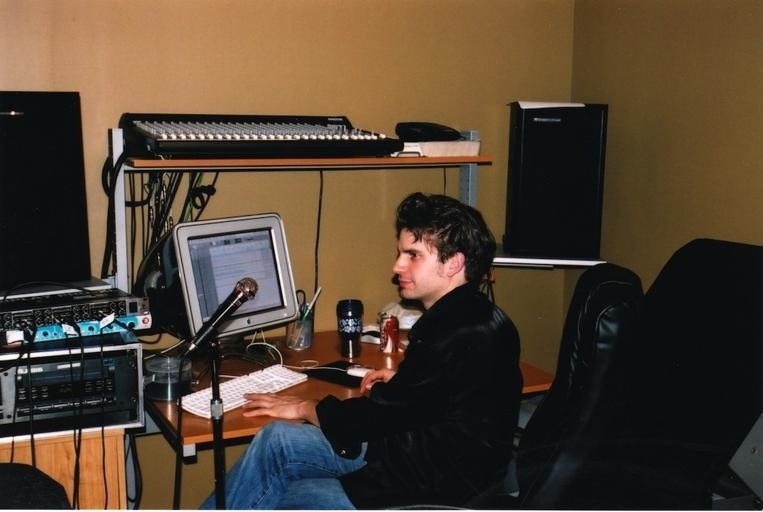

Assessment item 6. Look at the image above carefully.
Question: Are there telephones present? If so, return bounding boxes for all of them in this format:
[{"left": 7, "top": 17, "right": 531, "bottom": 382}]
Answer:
[{"left": 396, "top": 122, "right": 461, "bottom": 141}]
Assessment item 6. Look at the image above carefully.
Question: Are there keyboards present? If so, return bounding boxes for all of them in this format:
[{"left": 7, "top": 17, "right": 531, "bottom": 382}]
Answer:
[{"left": 176, "top": 364, "right": 308, "bottom": 420}]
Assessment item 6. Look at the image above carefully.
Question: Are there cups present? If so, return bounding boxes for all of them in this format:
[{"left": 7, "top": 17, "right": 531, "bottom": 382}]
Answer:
[
  {"left": 336, "top": 300, "right": 364, "bottom": 357},
  {"left": 287, "top": 321, "right": 312, "bottom": 351}
]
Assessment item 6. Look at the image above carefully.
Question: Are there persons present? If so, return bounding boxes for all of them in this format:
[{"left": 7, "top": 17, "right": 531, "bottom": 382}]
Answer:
[{"left": 186, "top": 191, "right": 525, "bottom": 510}]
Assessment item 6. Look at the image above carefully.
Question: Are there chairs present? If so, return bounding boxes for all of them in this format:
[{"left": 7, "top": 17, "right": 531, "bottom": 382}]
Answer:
[
  {"left": 380, "top": 264, "right": 644, "bottom": 509},
  {"left": 594, "top": 237, "right": 763, "bottom": 510}
]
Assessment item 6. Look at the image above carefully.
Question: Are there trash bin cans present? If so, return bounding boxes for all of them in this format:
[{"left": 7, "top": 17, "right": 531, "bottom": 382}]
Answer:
[{"left": 0, "top": 463, "right": 74, "bottom": 509}]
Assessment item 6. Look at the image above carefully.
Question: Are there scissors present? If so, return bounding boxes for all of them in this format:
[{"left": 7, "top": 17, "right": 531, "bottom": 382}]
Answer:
[{"left": 296, "top": 289, "right": 306, "bottom": 322}]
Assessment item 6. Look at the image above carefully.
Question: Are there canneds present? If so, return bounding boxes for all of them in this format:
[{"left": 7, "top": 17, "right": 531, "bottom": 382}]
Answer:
[{"left": 380, "top": 315, "right": 399, "bottom": 353}]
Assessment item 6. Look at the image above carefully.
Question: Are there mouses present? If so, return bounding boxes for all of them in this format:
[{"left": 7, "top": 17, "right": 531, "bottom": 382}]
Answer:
[{"left": 345, "top": 367, "right": 376, "bottom": 379}]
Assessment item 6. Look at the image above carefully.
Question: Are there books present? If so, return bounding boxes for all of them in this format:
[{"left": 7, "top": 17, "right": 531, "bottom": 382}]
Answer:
[{"left": 389, "top": 139, "right": 482, "bottom": 158}]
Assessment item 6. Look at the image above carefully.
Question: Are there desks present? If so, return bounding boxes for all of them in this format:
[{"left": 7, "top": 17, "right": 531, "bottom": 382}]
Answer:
[{"left": 141, "top": 324, "right": 555, "bottom": 509}]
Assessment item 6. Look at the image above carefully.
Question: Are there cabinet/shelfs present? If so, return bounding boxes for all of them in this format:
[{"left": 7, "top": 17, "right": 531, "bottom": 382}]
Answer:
[{"left": 0, "top": 429, "right": 129, "bottom": 509}]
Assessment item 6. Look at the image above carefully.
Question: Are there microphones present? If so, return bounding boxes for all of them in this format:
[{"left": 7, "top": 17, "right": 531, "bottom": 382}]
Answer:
[{"left": 183, "top": 276, "right": 259, "bottom": 358}]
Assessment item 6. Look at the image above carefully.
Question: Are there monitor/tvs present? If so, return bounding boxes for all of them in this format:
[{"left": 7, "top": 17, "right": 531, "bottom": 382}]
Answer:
[
  {"left": 134, "top": 211, "right": 301, "bottom": 342},
  {"left": 0, "top": 90, "right": 92, "bottom": 293}
]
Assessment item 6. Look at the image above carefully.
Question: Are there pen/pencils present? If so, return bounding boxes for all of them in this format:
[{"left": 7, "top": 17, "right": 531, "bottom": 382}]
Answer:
[{"left": 302, "top": 286, "right": 323, "bottom": 321}]
